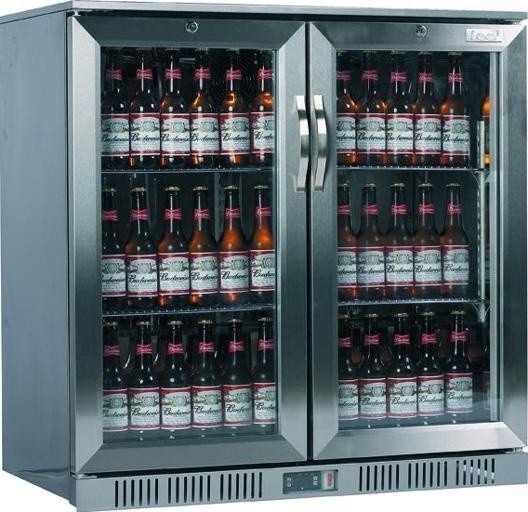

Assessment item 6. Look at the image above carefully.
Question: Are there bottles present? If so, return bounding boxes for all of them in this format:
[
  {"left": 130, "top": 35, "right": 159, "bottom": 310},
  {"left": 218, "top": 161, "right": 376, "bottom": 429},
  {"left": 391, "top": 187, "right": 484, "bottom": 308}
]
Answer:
[
  {"left": 439, "top": 184, "right": 471, "bottom": 300},
  {"left": 129, "top": 321, "right": 159, "bottom": 434},
  {"left": 123, "top": 188, "right": 158, "bottom": 311},
  {"left": 100, "top": 47, "right": 130, "bottom": 170},
  {"left": 416, "top": 311, "right": 445, "bottom": 420},
  {"left": 384, "top": 182, "right": 414, "bottom": 300},
  {"left": 102, "top": 319, "right": 129, "bottom": 440},
  {"left": 386, "top": 50, "right": 415, "bottom": 168},
  {"left": 158, "top": 47, "right": 192, "bottom": 170},
  {"left": 440, "top": 51, "right": 471, "bottom": 168},
  {"left": 102, "top": 187, "right": 127, "bottom": 312},
  {"left": 337, "top": 315, "right": 359, "bottom": 424},
  {"left": 481, "top": 71, "right": 490, "bottom": 166},
  {"left": 359, "top": 312, "right": 386, "bottom": 422},
  {"left": 337, "top": 179, "right": 358, "bottom": 300},
  {"left": 160, "top": 319, "right": 192, "bottom": 429},
  {"left": 222, "top": 319, "right": 255, "bottom": 430},
  {"left": 189, "top": 47, "right": 220, "bottom": 168},
  {"left": 129, "top": 46, "right": 162, "bottom": 171},
  {"left": 250, "top": 50, "right": 274, "bottom": 166},
  {"left": 220, "top": 187, "right": 252, "bottom": 309},
  {"left": 388, "top": 312, "right": 417, "bottom": 419},
  {"left": 250, "top": 185, "right": 276, "bottom": 305},
  {"left": 443, "top": 310, "right": 473, "bottom": 418},
  {"left": 337, "top": 51, "right": 357, "bottom": 166},
  {"left": 220, "top": 47, "right": 251, "bottom": 168},
  {"left": 158, "top": 185, "right": 190, "bottom": 308},
  {"left": 413, "top": 51, "right": 442, "bottom": 169},
  {"left": 412, "top": 184, "right": 442, "bottom": 300},
  {"left": 251, "top": 316, "right": 276, "bottom": 429},
  {"left": 357, "top": 182, "right": 387, "bottom": 301},
  {"left": 355, "top": 50, "right": 386, "bottom": 168},
  {"left": 187, "top": 186, "right": 219, "bottom": 310},
  {"left": 193, "top": 319, "right": 223, "bottom": 430}
]
